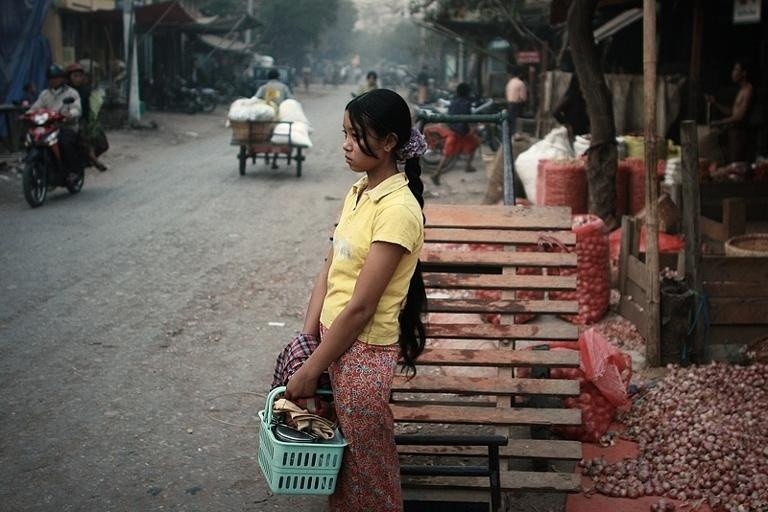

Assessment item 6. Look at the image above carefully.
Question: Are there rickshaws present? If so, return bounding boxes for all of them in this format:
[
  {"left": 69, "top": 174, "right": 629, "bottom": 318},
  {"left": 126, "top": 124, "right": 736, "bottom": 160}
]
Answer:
[{"left": 386, "top": 108, "right": 585, "bottom": 510}]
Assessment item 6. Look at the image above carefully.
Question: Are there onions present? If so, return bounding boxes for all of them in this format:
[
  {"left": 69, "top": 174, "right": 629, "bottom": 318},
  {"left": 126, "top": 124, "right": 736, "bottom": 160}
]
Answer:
[{"left": 470, "top": 213, "right": 611, "bottom": 326}]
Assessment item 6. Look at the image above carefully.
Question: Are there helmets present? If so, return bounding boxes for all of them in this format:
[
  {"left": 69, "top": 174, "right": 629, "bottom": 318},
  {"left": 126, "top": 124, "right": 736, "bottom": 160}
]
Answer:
[{"left": 46, "top": 64, "right": 83, "bottom": 77}]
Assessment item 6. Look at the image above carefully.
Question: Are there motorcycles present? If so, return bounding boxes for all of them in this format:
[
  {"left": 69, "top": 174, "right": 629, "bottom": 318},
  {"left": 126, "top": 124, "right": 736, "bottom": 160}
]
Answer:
[
  {"left": 404, "top": 74, "right": 501, "bottom": 172},
  {"left": 172, "top": 75, "right": 217, "bottom": 114},
  {"left": 142, "top": 80, "right": 200, "bottom": 114},
  {"left": 7, "top": 96, "right": 89, "bottom": 209}
]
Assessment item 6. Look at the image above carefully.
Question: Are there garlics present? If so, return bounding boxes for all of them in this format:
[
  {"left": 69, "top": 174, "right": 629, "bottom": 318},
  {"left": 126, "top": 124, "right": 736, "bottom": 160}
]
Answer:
[{"left": 515, "top": 340, "right": 767, "bottom": 512}]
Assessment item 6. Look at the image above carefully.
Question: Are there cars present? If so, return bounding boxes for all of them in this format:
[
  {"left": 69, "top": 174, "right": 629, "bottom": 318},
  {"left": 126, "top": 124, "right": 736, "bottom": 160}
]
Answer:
[{"left": 250, "top": 65, "right": 291, "bottom": 96}]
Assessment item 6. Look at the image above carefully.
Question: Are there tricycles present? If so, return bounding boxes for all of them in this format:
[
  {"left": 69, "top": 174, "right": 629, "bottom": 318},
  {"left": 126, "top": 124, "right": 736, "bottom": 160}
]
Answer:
[{"left": 235, "top": 120, "right": 308, "bottom": 179}]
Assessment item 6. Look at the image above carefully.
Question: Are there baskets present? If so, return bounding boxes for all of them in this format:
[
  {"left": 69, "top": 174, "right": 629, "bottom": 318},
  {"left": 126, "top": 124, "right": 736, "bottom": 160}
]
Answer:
[
  {"left": 258, "top": 386, "right": 348, "bottom": 495},
  {"left": 230, "top": 121, "right": 272, "bottom": 142},
  {"left": 725, "top": 233, "right": 768, "bottom": 258}
]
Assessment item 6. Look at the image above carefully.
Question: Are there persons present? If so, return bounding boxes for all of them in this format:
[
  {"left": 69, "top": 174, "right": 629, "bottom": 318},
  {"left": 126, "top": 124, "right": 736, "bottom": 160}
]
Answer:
[
  {"left": 356, "top": 68, "right": 380, "bottom": 96},
  {"left": 415, "top": 59, "right": 429, "bottom": 107},
  {"left": 66, "top": 63, "right": 110, "bottom": 171},
  {"left": 283, "top": 87, "right": 434, "bottom": 512},
  {"left": 550, "top": 77, "right": 589, "bottom": 144},
  {"left": 703, "top": 57, "right": 755, "bottom": 163},
  {"left": 504, "top": 66, "right": 528, "bottom": 130},
  {"left": 250, "top": 68, "right": 296, "bottom": 171},
  {"left": 429, "top": 80, "right": 479, "bottom": 187},
  {"left": 26, "top": 63, "right": 83, "bottom": 186},
  {"left": 301, "top": 59, "right": 312, "bottom": 96}
]
